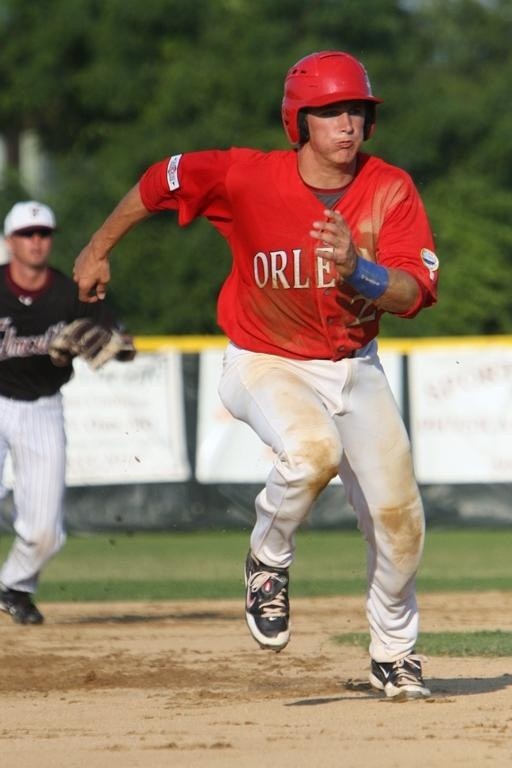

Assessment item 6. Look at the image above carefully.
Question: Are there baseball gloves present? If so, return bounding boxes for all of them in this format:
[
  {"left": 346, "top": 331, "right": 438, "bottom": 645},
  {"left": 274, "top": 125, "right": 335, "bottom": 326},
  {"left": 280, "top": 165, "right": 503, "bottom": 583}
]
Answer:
[{"left": 47, "top": 319, "right": 124, "bottom": 368}]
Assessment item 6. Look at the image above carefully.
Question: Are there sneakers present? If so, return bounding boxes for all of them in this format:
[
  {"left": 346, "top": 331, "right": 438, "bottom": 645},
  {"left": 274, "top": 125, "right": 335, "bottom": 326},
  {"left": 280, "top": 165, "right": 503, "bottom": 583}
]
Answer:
[
  {"left": 0, "top": 586, "right": 44, "bottom": 627},
  {"left": 366, "top": 653, "right": 430, "bottom": 700},
  {"left": 243, "top": 548, "right": 291, "bottom": 651}
]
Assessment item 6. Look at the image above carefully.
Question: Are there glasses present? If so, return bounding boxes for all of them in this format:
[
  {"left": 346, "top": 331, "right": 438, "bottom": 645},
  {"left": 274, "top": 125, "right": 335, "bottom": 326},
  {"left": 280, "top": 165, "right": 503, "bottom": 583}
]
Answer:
[{"left": 14, "top": 227, "right": 54, "bottom": 239}]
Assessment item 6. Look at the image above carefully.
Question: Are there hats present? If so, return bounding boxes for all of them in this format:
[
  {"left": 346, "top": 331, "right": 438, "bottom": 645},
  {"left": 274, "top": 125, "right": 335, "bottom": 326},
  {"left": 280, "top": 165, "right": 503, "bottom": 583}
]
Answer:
[
  {"left": 281, "top": 49, "right": 383, "bottom": 146},
  {"left": 4, "top": 201, "right": 58, "bottom": 240}
]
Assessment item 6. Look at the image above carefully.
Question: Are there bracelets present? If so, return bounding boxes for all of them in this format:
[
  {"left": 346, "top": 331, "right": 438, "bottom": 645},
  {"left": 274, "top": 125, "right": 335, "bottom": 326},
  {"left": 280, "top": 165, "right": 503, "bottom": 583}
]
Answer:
[{"left": 343, "top": 254, "right": 389, "bottom": 301}]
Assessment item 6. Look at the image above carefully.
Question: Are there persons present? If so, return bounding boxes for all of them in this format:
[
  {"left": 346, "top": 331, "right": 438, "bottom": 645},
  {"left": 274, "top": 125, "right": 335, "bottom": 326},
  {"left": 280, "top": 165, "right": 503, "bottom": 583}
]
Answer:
[
  {"left": 72, "top": 50, "right": 440, "bottom": 699},
  {"left": 0, "top": 202, "right": 136, "bottom": 626}
]
